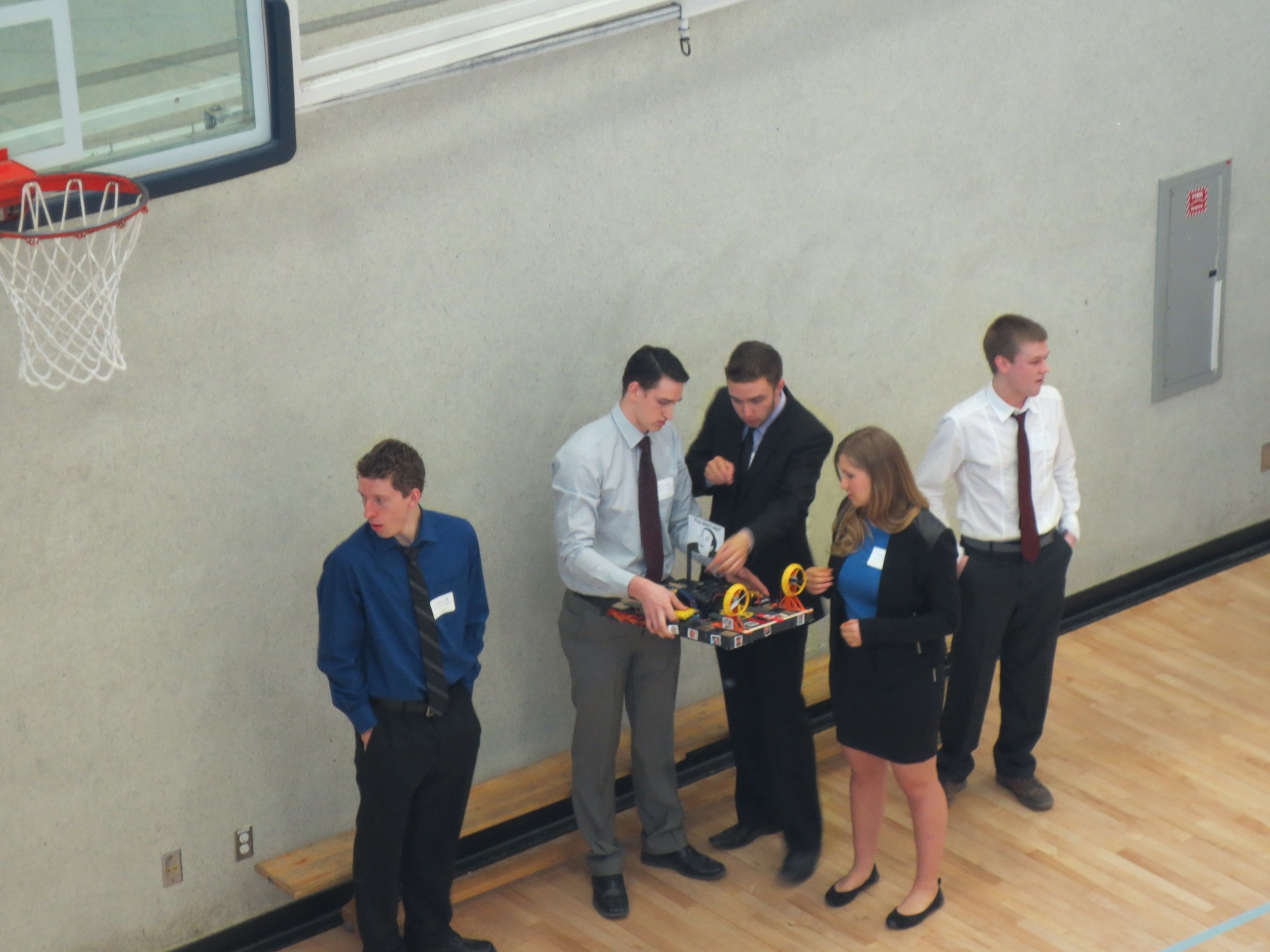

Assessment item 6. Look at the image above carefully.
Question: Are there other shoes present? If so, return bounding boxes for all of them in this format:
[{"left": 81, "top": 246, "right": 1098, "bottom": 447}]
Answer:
[
  {"left": 430, "top": 934, "right": 493, "bottom": 952},
  {"left": 886, "top": 879, "right": 945, "bottom": 929},
  {"left": 942, "top": 780, "right": 965, "bottom": 796},
  {"left": 997, "top": 774, "right": 1052, "bottom": 810},
  {"left": 825, "top": 865, "right": 880, "bottom": 907}
]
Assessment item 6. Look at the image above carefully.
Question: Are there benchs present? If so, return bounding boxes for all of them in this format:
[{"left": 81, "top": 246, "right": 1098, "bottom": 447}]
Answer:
[{"left": 255, "top": 656, "right": 829, "bottom": 926}]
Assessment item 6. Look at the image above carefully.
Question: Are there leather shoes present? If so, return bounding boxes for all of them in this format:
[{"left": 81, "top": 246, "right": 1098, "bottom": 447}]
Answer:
[
  {"left": 641, "top": 843, "right": 726, "bottom": 880},
  {"left": 710, "top": 822, "right": 782, "bottom": 848},
  {"left": 780, "top": 848, "right": 818, "bottom": 881},
  {"left": 592, "top": 873, "right": 630, "bottom": 917}
]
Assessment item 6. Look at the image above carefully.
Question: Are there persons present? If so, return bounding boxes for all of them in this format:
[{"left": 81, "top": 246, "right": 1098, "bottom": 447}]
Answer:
[
  {"left": 679, "top": 341, "right": 832, "bottom": 884},
  {"left": 920, "top": 315, "right": 1083, "bottom": 808},
  {"left": 316, "top": 439, "right": 497, "bottom": 952},
  {"left": 807, "top": 426, "right": 962, "bottom": 931},
  {"left": 550, "top": 345, "right": 769, "bottom": 920},
  {"left": 686, "top": 529, "right": 717, "bottom": 558}
]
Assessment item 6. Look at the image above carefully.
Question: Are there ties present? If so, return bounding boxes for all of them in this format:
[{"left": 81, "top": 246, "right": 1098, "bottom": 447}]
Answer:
[
  {"left": 737, "top": 429, "right": 755, "bottom": 486},
  {"left": 1012, "top": 411, "right": 1039, "bottom": 561},
  {"left": 637, "top": 436, "right": 663, "bottom": 582},
  {"left": 397, "top": 545, "right": 449, "bottom": 716}
]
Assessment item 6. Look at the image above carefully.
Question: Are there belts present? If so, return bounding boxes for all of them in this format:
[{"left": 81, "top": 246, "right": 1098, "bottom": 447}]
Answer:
[
  {"left": 962, "top": 529, "right": 1055, "bottom": 553},
  {"left": 376, "top": 685, "right": 463, "bottom": 717},
  {"left": 578, "top": 593, "right": 619, "bottom": 606}
]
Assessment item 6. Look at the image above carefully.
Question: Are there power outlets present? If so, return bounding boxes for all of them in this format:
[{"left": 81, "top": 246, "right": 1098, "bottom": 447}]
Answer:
[
  {"left": 234, "top": 824, "right": 254, "bottom": 862},
  {"left": 162, "top": 850, "right": 183, "bottom": 889}
]
